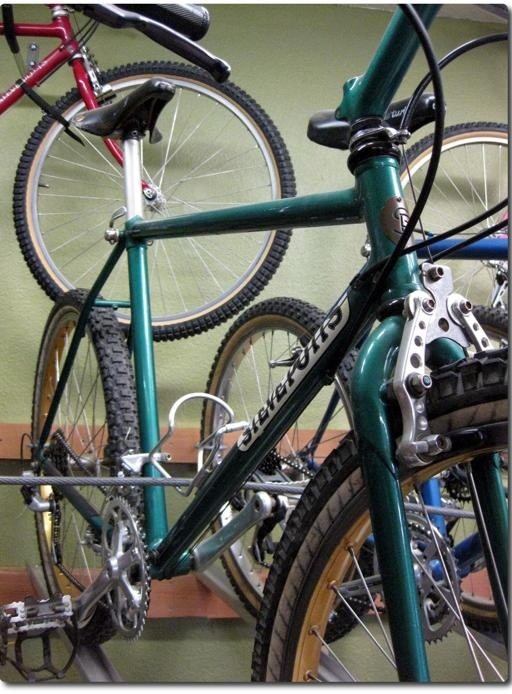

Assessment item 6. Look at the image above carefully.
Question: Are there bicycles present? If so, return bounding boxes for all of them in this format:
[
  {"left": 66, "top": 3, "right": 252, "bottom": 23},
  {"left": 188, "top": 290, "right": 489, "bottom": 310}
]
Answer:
[{"left": 2, "top": 4, "right": 510, "bottom": 683}]
